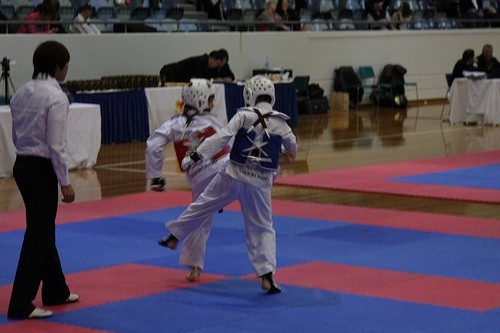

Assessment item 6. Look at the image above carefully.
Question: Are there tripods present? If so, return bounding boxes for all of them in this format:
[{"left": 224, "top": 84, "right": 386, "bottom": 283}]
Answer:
[{"left": 0, "top": 71, "right": 15, "bottom": 105}]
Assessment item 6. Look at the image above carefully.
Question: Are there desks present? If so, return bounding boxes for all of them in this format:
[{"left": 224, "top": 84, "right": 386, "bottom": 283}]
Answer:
[
  {"left": 0, "top": 103, "right": 102, "bottom": 178},
  {"left": 143, "top": 83, "right": 228, "bottom": 136},
  {"left": 447, "top": 78, "right": 500, "bottom": 125}
]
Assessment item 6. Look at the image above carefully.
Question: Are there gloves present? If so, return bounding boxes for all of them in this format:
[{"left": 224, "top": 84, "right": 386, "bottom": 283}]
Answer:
[
  {"left": 151, "top": 177, "right": 166, "bottom": 190},
  {"left": 179, "top": 149, "right": 204, "bottom": 171}
]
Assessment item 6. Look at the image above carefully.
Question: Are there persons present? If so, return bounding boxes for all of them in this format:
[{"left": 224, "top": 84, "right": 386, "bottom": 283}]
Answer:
[
  {"left": 18, "top": 0, "right": 500, "bottom": 37},
  {"left": 159, "top": 49, "right": 235, "bottom": 80},
  {"left": 5, "top": 40, "right": 79, "bottom": 319},
  {"left": 158, "top": 75, "right": 298, "bottom": 292},
  {"left": 450, "top": 44, "right": 500, "bottom": 87},
  {"left": 144, "top": 78, "right": 282, "bottom": 282}
]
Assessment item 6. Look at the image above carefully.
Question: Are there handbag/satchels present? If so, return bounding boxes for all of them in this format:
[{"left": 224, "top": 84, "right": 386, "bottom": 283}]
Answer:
[
  {"left": 304, "top": 97, "right": 330, "bottom": 112},
  {"left": 296, "top": 83, "right": 324, "bottom": 99}
]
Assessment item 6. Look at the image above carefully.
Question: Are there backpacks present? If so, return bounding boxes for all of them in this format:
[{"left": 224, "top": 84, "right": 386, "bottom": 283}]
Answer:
[
  {"left": 370, "top": 64, "right": 408, "bottom": 109},
  {"left": 334, "top": 66, "right": 361, "bottom": 92}
]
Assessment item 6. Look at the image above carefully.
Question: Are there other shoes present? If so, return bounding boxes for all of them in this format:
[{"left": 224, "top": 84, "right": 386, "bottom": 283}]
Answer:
[
  {"left": 7, "top": 308, "right": 53, "bottom": 319},
  {"left": 42, "top": 293, "right": 79, "bottom": 305}
]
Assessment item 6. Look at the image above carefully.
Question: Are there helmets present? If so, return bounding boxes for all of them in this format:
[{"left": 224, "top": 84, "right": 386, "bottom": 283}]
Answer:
[
  {"left": 243, "top": 75, "right": 276, "bottom": 107},
  {"left": 181, "top": 78, "right": 216, "bottom": 113}
]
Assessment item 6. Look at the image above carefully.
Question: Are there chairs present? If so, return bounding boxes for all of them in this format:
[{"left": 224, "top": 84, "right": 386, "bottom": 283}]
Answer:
[
  {"left": 212, "top": 0, "right": 462, "bottom": 31},
  {"left": 359, "top": 65, "right": 393, "bottom": 108},
  {"left": 97, "top": 6, "right": 198, "bottom": 32},
  {"left": 440, "top": 74, "right": 452, "bottom": 120},
  {"left": 294, "top": 76, "right": 313, "bottom": 113},
  {"left": 1, "top": 4, "right": 35, "bottom": 21},
  {"left": 60, "top": 6, "right": 76, "bottom": 30}
]
